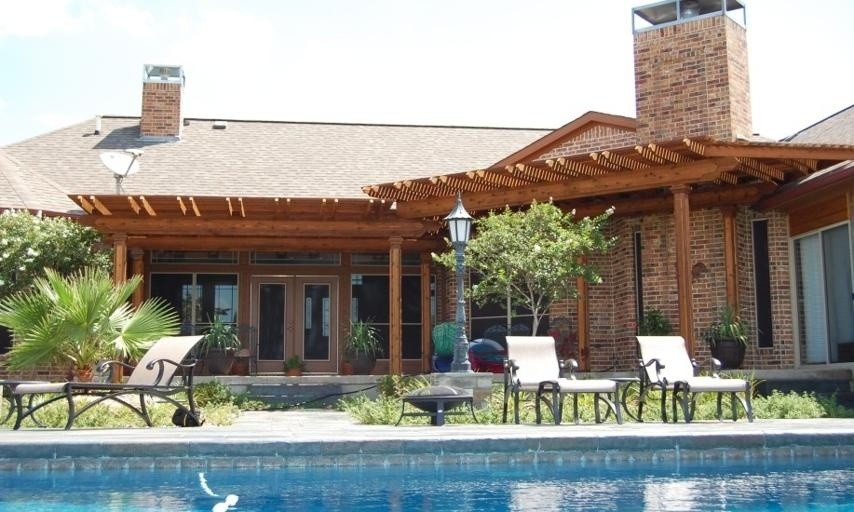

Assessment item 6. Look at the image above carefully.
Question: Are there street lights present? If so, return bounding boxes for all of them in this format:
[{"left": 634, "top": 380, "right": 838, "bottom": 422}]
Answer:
[{"left": 441, "top": 187, "right": 476, "bottom": 371}]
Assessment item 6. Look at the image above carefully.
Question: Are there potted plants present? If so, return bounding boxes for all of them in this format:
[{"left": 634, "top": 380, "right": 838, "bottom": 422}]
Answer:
[
  {"left": 195, "top": 311, "right": 241, "bottom": 374},
  {"left": 340, "top": 313, "right": 384, "bottom": 375},
  {"left": 282, "top": 356, "right": 308, "bottom": 376},
  {"left": 699, "top": 305, "right": 758, "bottom": 369}
]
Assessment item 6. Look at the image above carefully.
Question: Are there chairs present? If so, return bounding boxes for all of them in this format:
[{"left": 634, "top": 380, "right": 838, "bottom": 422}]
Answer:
[
  {"left": 640, "top": 470, "right": 853, "bottom": 512},
  {"left": 635, "top": 333, "right": 754, "bottom": 426},
  {"left": 508, "top": 477, "right": 623, "bottom": 512},
  {"left": 107, "top": 488, "right": 189, "bottom": 512},
  {"left": 12, "top": 333, "right": 206, "bottom": 431},
  {"left": 498, "top": 333, "right": 621, "bottom": 428}
]
